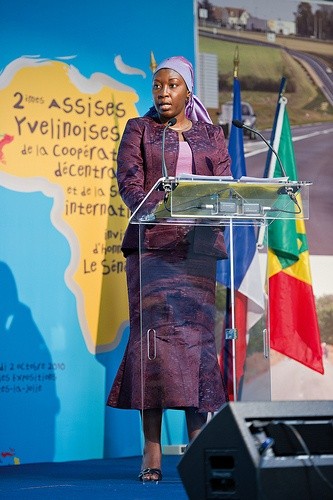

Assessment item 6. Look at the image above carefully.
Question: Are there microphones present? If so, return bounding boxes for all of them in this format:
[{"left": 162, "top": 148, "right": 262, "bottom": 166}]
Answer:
[
  {"left": 162, "top": 118, "right": 176, "bottom": 177},
  {"left": 232, "top": 120, "right": 286, "bottom": 177}
]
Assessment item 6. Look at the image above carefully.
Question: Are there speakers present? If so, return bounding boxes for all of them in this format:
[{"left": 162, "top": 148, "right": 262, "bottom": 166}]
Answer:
[{"left": 176, "top": 399, "right": 333, "bottom": 500}]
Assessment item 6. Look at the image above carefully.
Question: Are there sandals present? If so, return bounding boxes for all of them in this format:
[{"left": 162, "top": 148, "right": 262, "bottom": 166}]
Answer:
[{"left": 138, "top": 468, "right": 162, "bottom": 483}]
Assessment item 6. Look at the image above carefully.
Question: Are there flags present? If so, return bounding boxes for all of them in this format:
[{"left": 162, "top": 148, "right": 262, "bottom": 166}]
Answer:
[{"left": 217, "top": 70, "right": 325, "bottom": 403}]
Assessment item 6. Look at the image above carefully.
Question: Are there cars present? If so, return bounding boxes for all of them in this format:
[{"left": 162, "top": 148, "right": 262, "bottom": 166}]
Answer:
[{"left": 217, "top": 100, "right": 258, "bottom": 139}]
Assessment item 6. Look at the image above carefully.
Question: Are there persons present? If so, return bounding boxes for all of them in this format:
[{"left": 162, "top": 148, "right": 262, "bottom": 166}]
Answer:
[{"left": 104, "top": 54, "right": 233, "bottom": 483}]
